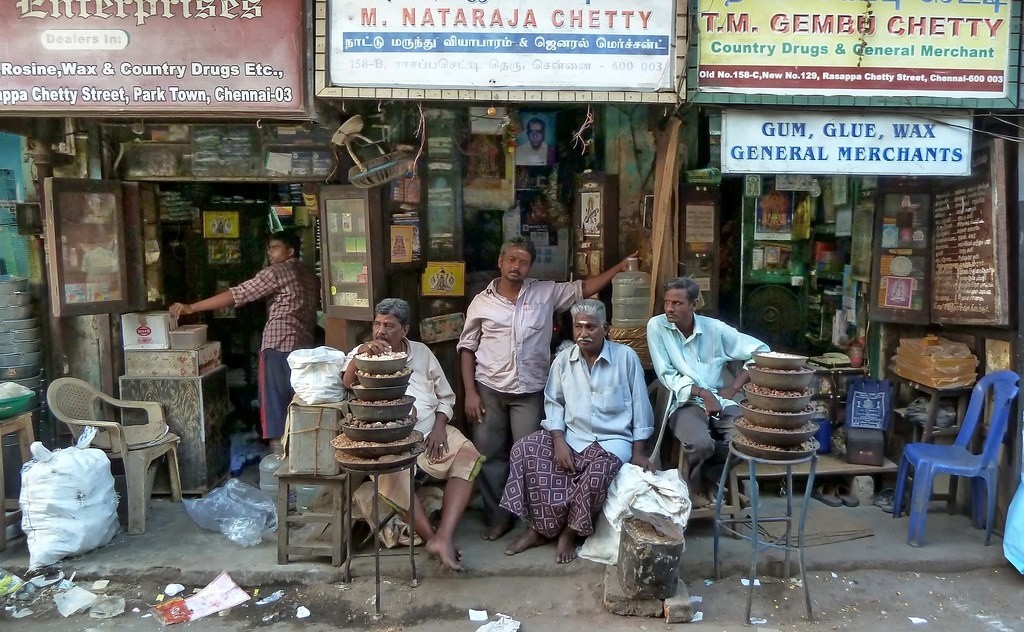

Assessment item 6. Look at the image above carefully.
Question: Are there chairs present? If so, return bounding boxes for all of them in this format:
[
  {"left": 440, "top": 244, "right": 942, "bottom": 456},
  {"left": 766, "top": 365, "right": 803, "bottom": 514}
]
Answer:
[
  {"left": 49, "top": 376, "right": 183, "bottom": 534},
  {"left": 643, "top": 379, "right": 675, "bottom": 468},
  {"left": 894, "top": 368, "right": 1019, "bottom": 547}
]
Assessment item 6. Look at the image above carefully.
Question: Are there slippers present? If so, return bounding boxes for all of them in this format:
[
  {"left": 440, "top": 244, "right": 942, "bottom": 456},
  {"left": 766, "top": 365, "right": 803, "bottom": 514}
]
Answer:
[
  {"left": 873, "top": 484, "right": 907, "bottom": 513},
  {"left": 810, "top": 480, "right": 860, "bottom": 507}
]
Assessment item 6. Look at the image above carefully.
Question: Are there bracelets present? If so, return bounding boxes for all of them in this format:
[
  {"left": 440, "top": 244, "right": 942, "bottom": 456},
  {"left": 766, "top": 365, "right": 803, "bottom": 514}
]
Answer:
[
  {"left": 552, "top": 435, "right": 563, "bottom": 442},
  {"left": 698, "top": 388, "right": 703, "bottom": 397}
]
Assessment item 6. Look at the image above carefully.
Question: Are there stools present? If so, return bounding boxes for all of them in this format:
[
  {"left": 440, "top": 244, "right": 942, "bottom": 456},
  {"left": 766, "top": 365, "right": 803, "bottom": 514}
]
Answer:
[
  {"left": 275, "top": 463, "right": 348, "bottom": 568},
  {"left": 0, "top": 412, "right": 37, "bottom": 551}
]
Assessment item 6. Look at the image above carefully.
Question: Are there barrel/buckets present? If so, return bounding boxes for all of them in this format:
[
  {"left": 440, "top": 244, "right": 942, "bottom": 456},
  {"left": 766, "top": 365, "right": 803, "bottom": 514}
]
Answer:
[
  {"left": 0, "top": 275, "right": 44, "bottom": 494},
  {"left": 812, "top": 417, "right": 831, "bottom": 455}
]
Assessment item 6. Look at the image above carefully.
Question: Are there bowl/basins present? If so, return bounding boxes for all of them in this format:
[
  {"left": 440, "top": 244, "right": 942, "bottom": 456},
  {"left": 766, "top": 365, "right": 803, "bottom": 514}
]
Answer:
[
  {"left": 0, "top": 392, "right": 36, "bottom": 419},
  {"left": 731, "top": 353, "right": 821, "bottom": 461},
  {"left": 329, "top": 352, "right": 431, "bottom": 470}
]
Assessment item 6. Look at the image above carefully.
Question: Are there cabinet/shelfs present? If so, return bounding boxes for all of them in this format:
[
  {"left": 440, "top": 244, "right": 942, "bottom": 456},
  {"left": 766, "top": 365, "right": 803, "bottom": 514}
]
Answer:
[
  {"left": 806, "top": 370, "right": 869, "bottom": 428},
  {"left": 342, "top": 457, "right": 423, "bottom": 621},
  {"left": 886, "top": 373, "right": 978, "bottom": 516},
  {"left": 48, "top": 121, "right": 382, "bottom": 320},
  {"left": 714, "top": 439, "right": 818, "bottom": 625},
  {"left": 742, "top": 178, "right": 932, "bottom": 365}
]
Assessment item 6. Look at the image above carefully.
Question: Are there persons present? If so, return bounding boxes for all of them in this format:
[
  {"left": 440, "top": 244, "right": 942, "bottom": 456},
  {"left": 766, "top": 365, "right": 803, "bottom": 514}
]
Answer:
[
  {"left": 504, "top": 299, "right": 657, "bottom": 564},
  {"left": 341, "top": 297, "right": 486, "bottom": 574},
  {"left": 169, "top": 231, "right": 318, "bottom": 454},
  {"left": 457, "top": 238, "right": 644, "bottom": 541},
  {"left": 646, "top": 277, "right": 771, "bottom": 507},
  {"left": 516, "top": 117, "right": 555, "bottom": 165}
]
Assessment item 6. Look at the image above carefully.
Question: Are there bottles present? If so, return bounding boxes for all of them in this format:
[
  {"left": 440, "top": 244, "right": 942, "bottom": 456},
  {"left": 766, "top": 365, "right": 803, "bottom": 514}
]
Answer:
[
  {"left": 611, "top": 258, "right": 651, "bottom": 328},
  {"left": 258, "top": 440, "right": 326, "bottom": 514},
  {"left": 849, "top": 342, "right": 863, "bottom": 368}
]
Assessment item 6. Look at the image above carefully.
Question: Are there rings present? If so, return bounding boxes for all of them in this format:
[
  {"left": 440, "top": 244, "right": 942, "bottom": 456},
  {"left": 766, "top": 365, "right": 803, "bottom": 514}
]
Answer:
[
  {"left": 367, "top": 344, "right": 372, "bottom": 348},
  {"left": 439, "top": 444, "right": 444, "bottom": 448}
]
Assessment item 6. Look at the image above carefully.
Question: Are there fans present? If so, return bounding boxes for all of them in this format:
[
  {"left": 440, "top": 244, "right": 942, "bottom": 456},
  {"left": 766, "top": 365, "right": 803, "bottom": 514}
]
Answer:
[
  {"left": 331, "top": 112, "right": 410, "bottom": 189},
  {"left": 744, "top": 285, "right": 802, "bottom": 341}
]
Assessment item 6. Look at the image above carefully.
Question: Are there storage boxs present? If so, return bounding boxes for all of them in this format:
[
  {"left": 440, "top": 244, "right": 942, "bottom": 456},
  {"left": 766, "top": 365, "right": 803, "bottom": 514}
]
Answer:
[
  {"left": 123, "top": 309, "right": 178, "bottom": 350},
  {"left": 125, "top": 341, "right": 221, "bottom": 378},
  {"left": 169, "top": 322, "right": 209, "bottom": 349}
]
